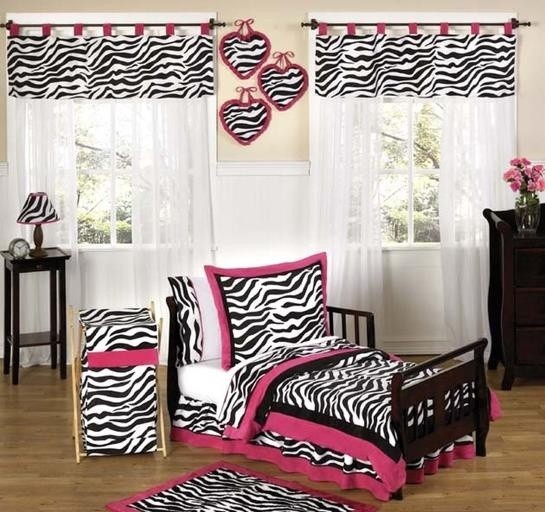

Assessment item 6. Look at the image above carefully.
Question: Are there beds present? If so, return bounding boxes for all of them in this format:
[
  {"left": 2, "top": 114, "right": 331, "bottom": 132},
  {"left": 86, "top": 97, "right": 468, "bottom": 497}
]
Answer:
[{"left": 167, "top": 251, "right": 491, "bottom": 501}]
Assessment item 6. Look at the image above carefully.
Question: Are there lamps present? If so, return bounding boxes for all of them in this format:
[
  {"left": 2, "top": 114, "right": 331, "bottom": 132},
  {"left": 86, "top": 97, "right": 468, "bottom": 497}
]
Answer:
[{"left": 16, "top": 192, "right": 61, "bottom": 258}]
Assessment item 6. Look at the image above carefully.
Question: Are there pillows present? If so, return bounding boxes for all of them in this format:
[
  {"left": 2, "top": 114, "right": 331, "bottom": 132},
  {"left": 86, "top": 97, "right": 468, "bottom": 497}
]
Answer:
[
  {"left": 202, "top": 252, "right": 328, "bottom": 370},
  {"left": 169, "top": 277, "right": 220, "bottom": 366}
]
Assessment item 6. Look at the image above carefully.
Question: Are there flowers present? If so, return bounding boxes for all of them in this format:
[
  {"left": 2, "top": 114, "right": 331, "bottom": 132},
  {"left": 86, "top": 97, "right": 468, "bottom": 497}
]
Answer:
[{"left": 501, "top": 155, "right": 545, "bottom": 220}]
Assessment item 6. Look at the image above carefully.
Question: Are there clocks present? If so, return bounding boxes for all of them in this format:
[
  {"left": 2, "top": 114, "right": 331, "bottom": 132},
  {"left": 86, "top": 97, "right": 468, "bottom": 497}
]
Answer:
[{"left": 9, "top": 237, "right": 30, "bottom": 257}]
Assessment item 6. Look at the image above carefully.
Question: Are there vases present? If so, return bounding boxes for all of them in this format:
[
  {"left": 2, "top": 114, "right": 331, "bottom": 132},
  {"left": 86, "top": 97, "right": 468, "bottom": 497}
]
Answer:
[{"left": 513, "top": 200, "right": 541, "bottom": 236}]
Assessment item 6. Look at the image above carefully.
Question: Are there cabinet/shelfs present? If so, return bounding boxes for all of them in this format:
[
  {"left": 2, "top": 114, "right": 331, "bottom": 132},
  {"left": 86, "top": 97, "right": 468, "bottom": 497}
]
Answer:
[{"left": 485, "top": 203, "right": 545, "bottom": 388}]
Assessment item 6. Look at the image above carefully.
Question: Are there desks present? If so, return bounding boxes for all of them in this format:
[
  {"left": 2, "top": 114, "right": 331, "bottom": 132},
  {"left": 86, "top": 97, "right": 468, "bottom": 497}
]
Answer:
[{"left": 1, "top": 247, "right": 73, "bottom": 386}]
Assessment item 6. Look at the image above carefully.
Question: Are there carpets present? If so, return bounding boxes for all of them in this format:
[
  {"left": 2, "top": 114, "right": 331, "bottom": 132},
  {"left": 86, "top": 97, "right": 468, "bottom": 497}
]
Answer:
[{"left": 103, "top": 458, "right": 378, "bottom": 512}]
